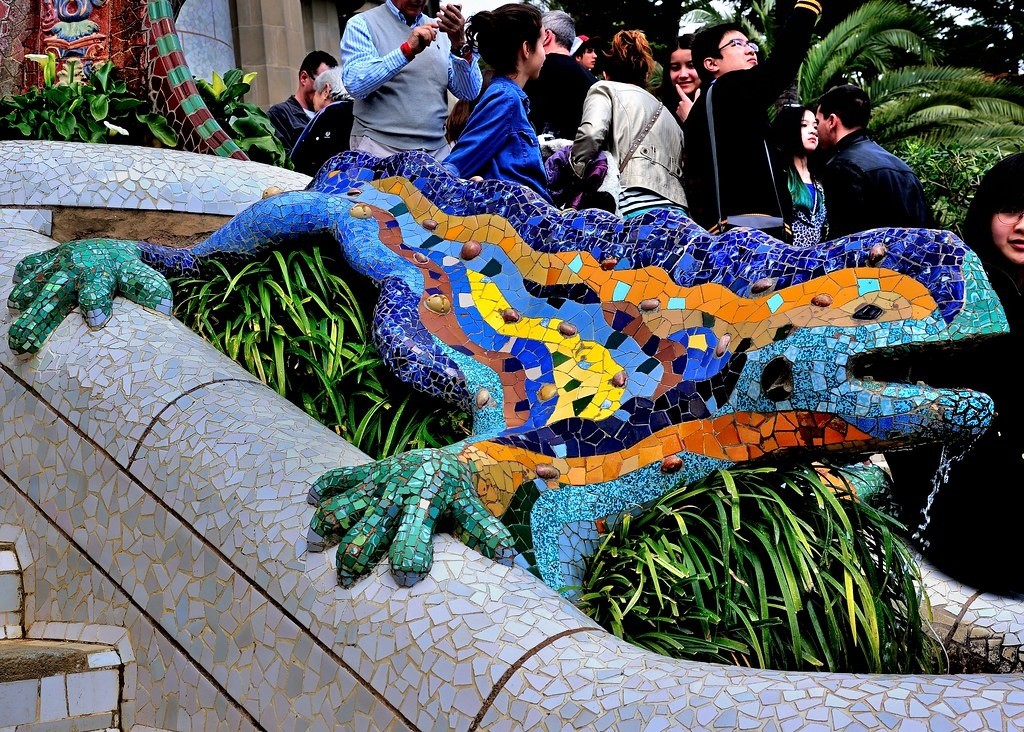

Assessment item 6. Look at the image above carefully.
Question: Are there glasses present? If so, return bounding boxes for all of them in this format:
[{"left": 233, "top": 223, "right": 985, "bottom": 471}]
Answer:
[
  {"left": 783, "top": 104, "right": 803, "bottom": 107},
  {"left": 998, "top": 206, "right": 1024, "bottom": 225},
  {"left": 719, "top": 39, "right": 759, "bottom": 52}
]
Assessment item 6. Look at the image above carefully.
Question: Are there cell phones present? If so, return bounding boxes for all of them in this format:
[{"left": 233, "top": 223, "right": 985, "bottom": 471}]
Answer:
[{"left": 439, "top": 5, "right": 460, "bottom": 32}]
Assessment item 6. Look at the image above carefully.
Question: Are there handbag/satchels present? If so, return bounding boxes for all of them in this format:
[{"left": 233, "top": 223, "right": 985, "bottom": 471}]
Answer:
[{"left": 717, "top": 216, "right": 785, "bottom": 239}]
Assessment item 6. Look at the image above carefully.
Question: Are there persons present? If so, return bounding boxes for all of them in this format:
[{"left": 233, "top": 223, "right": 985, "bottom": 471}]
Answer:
[
  {"left": 341, "top": 0, "right": 483, "bottom": 163},
  {"left": 266, "top": 50, "right": 354, "bottom": 177},
  {"left": 442, "top": 4, "right": 688, "bottom": 220},
  {"left": 911, "top": 153, "right": 1024, "bottom": 599},
  {"left": 651, "top": 0, "right": 828, "bottom": 245},
  {"left": 816, "top": 85, "right": 935, "bottom": 538}
]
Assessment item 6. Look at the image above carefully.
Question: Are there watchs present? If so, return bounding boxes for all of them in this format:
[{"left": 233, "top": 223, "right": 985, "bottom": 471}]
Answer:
[{"left": 451, "top": 44, "right": 469, "bottom": 56}]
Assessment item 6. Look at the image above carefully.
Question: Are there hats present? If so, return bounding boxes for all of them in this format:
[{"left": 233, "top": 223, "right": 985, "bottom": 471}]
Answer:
[{"left": 570, "top": 35, "right": 601, "bottom": 57}]
